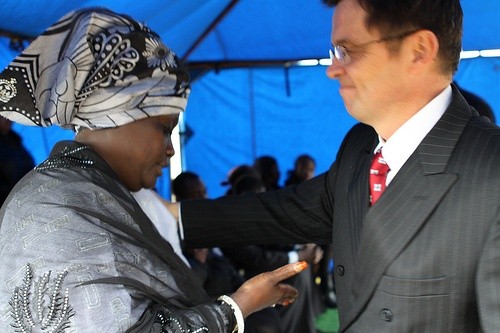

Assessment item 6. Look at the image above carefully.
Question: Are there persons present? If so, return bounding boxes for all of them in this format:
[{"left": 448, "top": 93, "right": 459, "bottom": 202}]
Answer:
[
  {"left": 0, "top": 7, "right": 307, "bottom": 333},
  {"left": 160, "top": 0, "right": 500, "bottom": 333},
  {"left": 0, "top": 116, "right": 338, "bottom": 333}
]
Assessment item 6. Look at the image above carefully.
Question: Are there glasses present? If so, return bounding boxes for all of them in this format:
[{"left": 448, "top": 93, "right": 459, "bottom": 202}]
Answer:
[{"left": 329, "top": 30, "right": 418, "bottom": 66}]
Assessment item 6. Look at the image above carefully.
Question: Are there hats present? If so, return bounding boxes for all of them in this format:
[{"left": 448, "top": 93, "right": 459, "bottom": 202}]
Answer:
[
  {"left": 221, "top": 166, "right": 254, "bottom": 185},
  {"left": 236, "top": 177, "right": 266, "bottom": 193}
]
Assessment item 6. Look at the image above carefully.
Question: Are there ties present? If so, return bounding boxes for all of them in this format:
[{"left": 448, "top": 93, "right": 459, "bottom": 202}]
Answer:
[{"left": 368, "top": 148, "right": 390, "bottom": 207}]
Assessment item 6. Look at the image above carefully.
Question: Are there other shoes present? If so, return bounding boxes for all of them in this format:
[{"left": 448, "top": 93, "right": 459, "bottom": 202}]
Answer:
[{"left": 324, "top": 290, "right": 337, "bottom": 307}]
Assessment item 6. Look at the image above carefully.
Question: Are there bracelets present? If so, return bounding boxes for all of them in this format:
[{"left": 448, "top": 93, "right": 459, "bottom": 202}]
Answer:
[{"left": 217, "top": 295, "right": 245, "bottom": 333}]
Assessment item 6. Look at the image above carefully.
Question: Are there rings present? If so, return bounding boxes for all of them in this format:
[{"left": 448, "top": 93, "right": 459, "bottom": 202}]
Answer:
[{"left": 271, "top": 303, "right": 276, "bottom": 308}]
